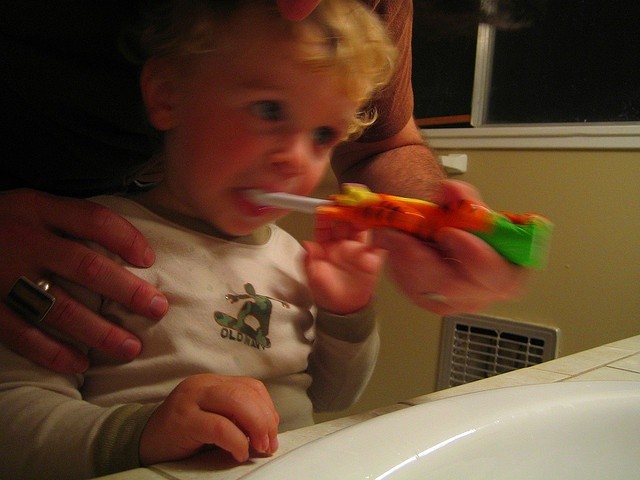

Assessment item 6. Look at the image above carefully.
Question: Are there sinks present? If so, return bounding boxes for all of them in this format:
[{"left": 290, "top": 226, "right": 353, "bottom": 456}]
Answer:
[{"left": 248, "top": 378, "right": 639, "bottom": 480}]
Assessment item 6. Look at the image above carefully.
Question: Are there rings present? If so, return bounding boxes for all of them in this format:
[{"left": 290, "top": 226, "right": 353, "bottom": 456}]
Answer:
[{"left": 4, "top": 273, "right": 55, "bottom": 326}]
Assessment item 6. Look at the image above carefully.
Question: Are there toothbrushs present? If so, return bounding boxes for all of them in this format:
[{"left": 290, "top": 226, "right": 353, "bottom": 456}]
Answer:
[{"left": 244, "top": 186, "right": 555, "bottom": 270}]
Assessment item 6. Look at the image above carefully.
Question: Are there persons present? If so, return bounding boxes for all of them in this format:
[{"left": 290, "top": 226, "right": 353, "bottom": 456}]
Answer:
[
  {"left": 0, "top": 0, "right": 532, "bottom": 377},
  {"left": 0, "top": 0, "right": 392, "bottom": 479}
]
